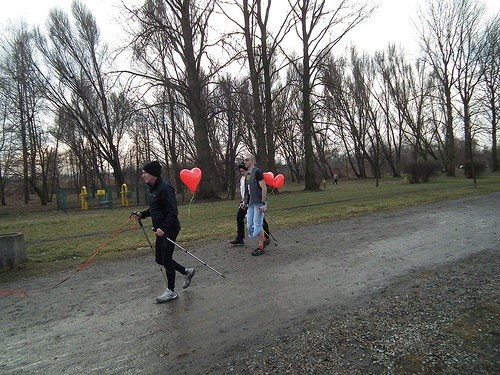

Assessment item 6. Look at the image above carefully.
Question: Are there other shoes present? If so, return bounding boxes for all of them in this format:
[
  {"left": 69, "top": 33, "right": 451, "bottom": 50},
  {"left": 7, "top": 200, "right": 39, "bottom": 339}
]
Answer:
[
  {"left": 264, "top": 239, "right": 270, "bottom": 248},
  {"left": 251, "top": 248, "right": 264, "bottom": 255},
  {"left": 230, "top": 237, "right": 245, "bottom": 244}
]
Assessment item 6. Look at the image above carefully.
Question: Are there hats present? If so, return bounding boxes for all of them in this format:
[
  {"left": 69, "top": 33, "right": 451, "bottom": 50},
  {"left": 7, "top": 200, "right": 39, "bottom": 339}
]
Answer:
[
  {"left": 245, "top": 154, "right": 255, "bottom": 160},
  {"left": 142, "top": 161, "right": 162, "bottom": 177},
  {"left": 239, "top": 162, "right": 248, "bottom": 171}
]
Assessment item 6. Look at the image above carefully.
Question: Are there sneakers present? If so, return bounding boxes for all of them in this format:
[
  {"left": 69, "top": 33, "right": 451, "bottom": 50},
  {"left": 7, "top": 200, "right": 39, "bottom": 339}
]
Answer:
[
  {"left": 156, "top": 287, "right": 178, "bottom": 303},
  {"left": 182, "top": 267, "right": 195, "bottom": 289}
]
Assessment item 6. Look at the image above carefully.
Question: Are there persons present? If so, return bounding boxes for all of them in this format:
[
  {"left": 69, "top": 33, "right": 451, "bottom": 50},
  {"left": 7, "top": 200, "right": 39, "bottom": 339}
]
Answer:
[
  {"left": 333, "top": 173, "right": 338, "bottom": 185},
  {"left": 133, "top": 161, "right": 195, "bottom": 302},
  {"left": 230, "top": 154, "right": 270, "bottom": 255}
]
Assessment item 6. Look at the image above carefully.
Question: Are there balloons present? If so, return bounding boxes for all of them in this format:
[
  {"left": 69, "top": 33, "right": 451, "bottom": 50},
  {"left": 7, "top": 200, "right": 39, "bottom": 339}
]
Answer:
[
  {"left": 263, "top": 172, "right": 274, "bottom": 185},
  {"left": 273, "top": 174, "right": 284, "bottom": 188},
  {"left": 180, "top": 168, "right": 202, "bottom": 193}
]
